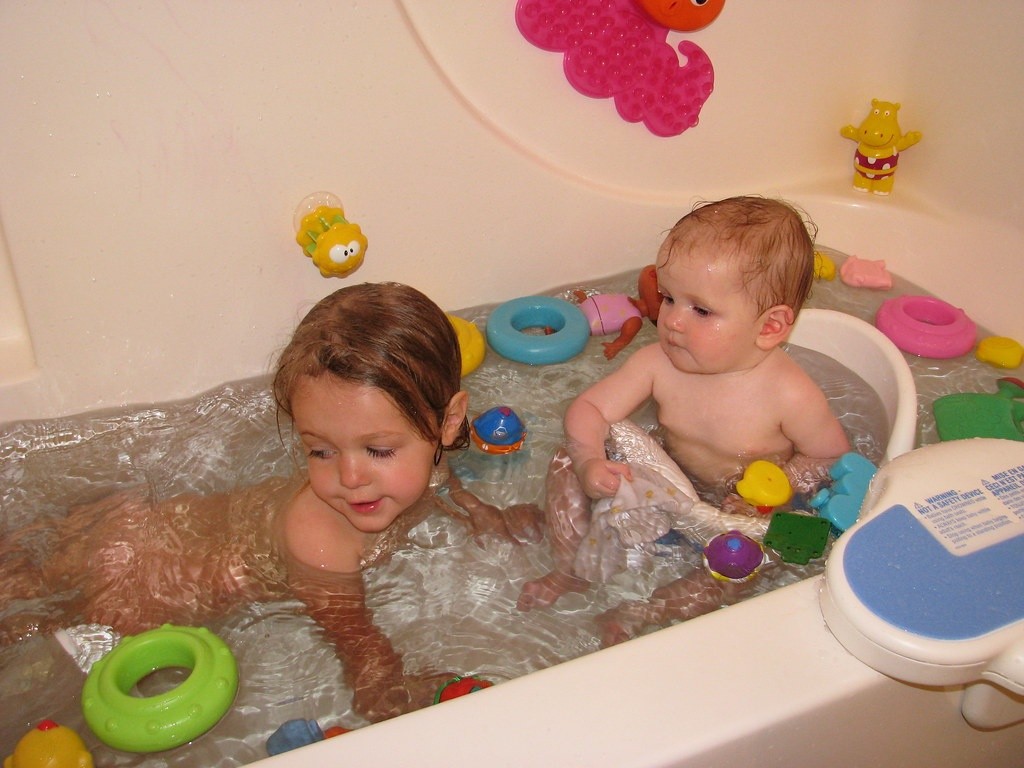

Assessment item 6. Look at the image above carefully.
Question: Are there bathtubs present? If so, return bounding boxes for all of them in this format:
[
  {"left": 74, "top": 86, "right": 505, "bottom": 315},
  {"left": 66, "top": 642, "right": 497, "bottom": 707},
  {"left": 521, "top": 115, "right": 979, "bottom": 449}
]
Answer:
[{"left": 0, "top": 199, "right": 1024, "bottom": 768}]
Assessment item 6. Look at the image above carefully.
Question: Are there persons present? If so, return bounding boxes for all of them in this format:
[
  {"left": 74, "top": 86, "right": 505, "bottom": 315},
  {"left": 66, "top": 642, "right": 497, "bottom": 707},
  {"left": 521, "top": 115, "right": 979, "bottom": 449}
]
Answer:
[
  {"left": 565, "top": 196, "right": 849, "bottom": 498},
  {"left": 276, "top": 282, "right": 472, "bottom": 573}
]
